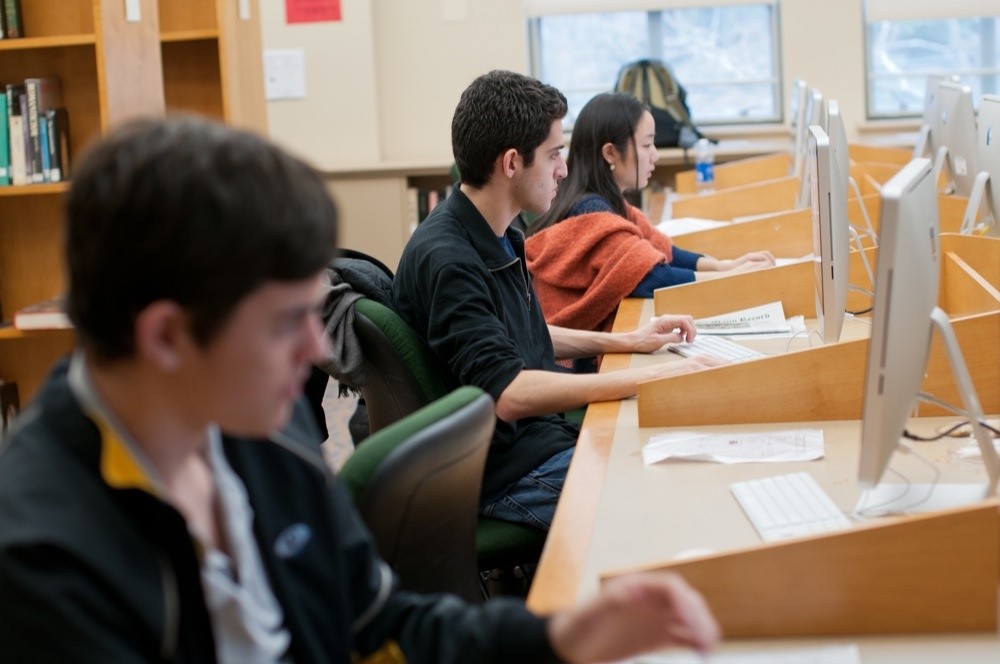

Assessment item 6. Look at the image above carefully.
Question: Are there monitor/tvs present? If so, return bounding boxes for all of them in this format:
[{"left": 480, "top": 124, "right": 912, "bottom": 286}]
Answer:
[{"left": 791, "top": 71, "right": 999, "bottom": 499}]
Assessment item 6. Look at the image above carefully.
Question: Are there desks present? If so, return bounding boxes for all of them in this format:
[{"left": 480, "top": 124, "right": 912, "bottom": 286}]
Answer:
[{"left": 526, "top": 141, "right": 1000, "bottom": 662}]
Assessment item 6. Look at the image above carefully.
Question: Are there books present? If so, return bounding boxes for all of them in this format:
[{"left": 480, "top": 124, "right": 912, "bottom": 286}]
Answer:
[
  {"left": 14, "top": 300, "right": 76, "bottom": 330},
  {"left": 0, "top": 76, "right": 72, "bottom": 185},
  {"left": 0, "top": 380, "right": 20, "bottom": 429},
  {"left": 0, "top": 0, "right": 21, "bottom": 37}
]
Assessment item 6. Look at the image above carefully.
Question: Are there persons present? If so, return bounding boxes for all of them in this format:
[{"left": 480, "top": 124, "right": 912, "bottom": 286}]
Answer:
[
  {"left": 388, "top": 69, "right": 726, "bottom": 528},
  {"left": 614, "top": 59, "right": 705, "bottom": 143},
  {"left": 0, "top": 113, "right": 719, "bottom": 663},
  {"left": 518, "top": 91, "right": 774, "bottom": 298}
]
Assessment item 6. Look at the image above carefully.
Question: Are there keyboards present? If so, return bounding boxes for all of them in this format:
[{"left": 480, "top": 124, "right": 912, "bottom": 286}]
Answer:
[
  {"left": 670, "top": 336, "right": 767, "bottom": 365},
  {"left": 731, "top": 472, "right": 854, "bottom": 545}
]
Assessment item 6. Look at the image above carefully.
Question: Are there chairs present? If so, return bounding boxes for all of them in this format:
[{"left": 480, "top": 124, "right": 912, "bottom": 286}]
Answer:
[
  {"left": 331, "top": 247, "right": 546, "bottom": 605},
  {"left": 331, "top": 385, "right": 489, "bottom": 611}
]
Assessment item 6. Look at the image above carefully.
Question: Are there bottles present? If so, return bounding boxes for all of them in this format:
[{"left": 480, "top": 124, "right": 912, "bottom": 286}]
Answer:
[{"left": 693, "top": 138, "right": 717, "bottom": 196}]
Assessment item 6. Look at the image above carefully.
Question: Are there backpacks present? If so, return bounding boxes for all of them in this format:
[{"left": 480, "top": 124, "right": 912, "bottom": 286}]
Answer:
[{"left": 616, "top": 60, "right": 717, "bottom": 149}]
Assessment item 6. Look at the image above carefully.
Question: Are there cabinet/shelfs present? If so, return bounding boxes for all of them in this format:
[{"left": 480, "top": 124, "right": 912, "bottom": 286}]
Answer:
[{"left": 0, "top": 0, "right": 267, "bottom": 443}]
